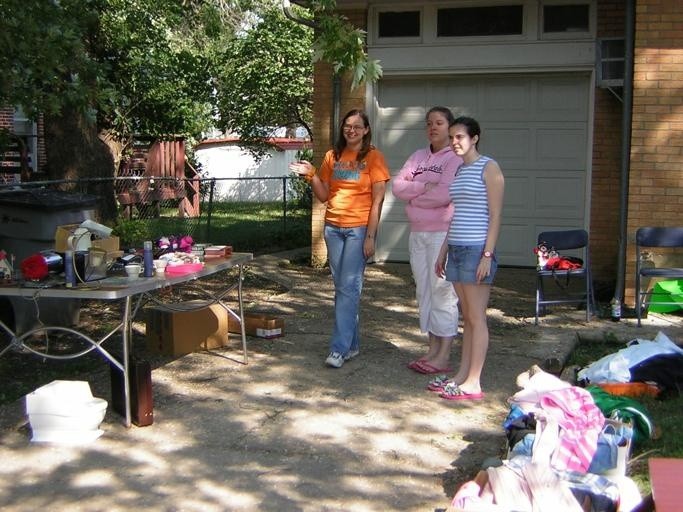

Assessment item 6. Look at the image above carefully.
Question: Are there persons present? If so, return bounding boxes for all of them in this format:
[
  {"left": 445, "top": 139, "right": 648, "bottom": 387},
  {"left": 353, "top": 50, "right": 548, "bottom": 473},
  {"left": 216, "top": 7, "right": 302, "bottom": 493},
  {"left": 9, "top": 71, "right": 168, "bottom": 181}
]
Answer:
[
  {"left": 392, "top": 105, "right": 461, "bottom": 373},
  {"left": 425, "top": 114, "right": 505, "bottom": 400},
  {"left": 289, "top": 109, "right": 390, "bottom": 367}
]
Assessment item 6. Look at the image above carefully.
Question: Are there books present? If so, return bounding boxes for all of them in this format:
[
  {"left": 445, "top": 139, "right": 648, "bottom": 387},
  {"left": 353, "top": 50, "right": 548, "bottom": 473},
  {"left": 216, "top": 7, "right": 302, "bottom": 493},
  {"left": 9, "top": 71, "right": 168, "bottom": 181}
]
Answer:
[{"left": 190, "top": 240, "right": 232, "bottom": 258}]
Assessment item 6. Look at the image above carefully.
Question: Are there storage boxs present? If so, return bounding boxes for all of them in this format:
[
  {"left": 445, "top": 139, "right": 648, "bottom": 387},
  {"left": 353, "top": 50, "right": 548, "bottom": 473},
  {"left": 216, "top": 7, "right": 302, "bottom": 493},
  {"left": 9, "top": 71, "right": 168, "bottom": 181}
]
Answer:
[
  {"left": 228, "top": 310, "right": 286, "bottom": 340},
  {"left": 140, "top": 299, "right": 229, "bottom": 360},
  {"left": 54, "top": 223, "right": 120, "bottom": 264}
]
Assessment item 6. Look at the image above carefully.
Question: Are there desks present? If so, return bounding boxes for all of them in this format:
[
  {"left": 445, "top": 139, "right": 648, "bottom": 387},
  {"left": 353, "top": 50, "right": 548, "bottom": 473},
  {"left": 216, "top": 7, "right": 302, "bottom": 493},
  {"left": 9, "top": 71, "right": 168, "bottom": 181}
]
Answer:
[{"left": 0, "top": 249, "right": 253, "bottom": 427}]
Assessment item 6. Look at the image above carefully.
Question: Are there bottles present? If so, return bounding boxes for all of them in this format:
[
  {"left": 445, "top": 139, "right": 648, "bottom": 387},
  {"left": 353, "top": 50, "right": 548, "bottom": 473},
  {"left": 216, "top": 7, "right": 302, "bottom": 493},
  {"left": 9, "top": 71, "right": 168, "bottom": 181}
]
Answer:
[
  {"left": 64, "top": 249, "right": 77, "bottom": 289},
  {"left": 142, "top": 241, "right": 154, "bottom": 278},
  {"left": 610, "top": 296, "right": 622, "bottom": 324}
]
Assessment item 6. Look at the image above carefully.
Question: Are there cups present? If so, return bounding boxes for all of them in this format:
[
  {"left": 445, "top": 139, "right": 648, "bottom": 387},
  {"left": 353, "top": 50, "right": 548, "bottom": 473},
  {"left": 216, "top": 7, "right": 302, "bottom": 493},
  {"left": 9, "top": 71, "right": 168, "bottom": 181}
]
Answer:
[
  {"left": 124, "top": 264, "right": 147, "bottom": 280},
  {"left": 151, "top": 259, "right": 169, "bottom": 274}
]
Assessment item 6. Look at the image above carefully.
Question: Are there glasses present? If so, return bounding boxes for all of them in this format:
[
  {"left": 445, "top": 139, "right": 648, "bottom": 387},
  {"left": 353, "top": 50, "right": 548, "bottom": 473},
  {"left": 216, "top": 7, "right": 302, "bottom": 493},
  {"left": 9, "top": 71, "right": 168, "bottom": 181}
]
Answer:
[{"left": 343, "top": 125, "right": 363, "bottom": 131}]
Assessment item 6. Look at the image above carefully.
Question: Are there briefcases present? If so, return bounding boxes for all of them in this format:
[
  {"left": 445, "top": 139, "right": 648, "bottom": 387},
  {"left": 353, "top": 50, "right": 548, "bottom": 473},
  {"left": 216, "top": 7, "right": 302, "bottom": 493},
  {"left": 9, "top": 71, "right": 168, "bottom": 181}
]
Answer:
[{"left": 110, "top": 358, "right": 154, "bottom": 427}]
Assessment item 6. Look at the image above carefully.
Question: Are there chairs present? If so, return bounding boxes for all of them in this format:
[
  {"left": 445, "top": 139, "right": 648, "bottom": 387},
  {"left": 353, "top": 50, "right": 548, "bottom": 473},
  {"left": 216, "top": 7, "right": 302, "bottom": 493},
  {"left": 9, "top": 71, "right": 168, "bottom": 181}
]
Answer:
[
  {"left": 528, "top": 227, "right": 596, "bottom": 324},
  {"left": 634, "top": 224, "right": 683, "bottom": 329}
]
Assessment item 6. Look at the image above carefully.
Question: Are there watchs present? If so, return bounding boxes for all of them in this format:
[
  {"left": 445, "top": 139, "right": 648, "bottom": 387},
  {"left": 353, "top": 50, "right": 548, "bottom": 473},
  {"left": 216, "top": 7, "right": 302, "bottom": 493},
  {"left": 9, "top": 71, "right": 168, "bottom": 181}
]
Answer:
[{"left": 482, "top": 250, "right": 494, "bottom": 261}]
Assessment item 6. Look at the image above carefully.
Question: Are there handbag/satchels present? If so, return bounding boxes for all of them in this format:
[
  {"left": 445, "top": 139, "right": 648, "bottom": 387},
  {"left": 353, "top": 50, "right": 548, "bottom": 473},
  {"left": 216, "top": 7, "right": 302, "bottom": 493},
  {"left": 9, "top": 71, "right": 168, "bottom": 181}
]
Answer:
[{"left": 546, "top": 255, "right": 583, "bottom": 270}]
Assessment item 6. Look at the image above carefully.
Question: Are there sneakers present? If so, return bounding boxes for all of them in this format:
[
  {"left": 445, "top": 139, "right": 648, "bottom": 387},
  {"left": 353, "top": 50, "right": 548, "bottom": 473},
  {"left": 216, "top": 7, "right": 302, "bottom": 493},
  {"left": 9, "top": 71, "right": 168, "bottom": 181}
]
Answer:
[{"left": 325, "top": 350, "right": 360, "bottom": 368}]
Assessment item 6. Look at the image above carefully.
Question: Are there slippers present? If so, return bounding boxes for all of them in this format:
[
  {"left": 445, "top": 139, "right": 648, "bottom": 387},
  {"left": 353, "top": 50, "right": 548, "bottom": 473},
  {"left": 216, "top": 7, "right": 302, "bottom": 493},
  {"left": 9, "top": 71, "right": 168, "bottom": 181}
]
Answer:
[{"left": 408, "top": 356, "right": 483, "bottom": 399}]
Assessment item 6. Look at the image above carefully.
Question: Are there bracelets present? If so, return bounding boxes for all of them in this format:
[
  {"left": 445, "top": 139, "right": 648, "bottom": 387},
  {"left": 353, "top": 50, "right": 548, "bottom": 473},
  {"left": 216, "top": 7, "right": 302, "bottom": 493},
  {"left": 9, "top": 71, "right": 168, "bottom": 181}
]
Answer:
[{"left": 305, "top": 163, "right": 317, "bottom": 182}]
